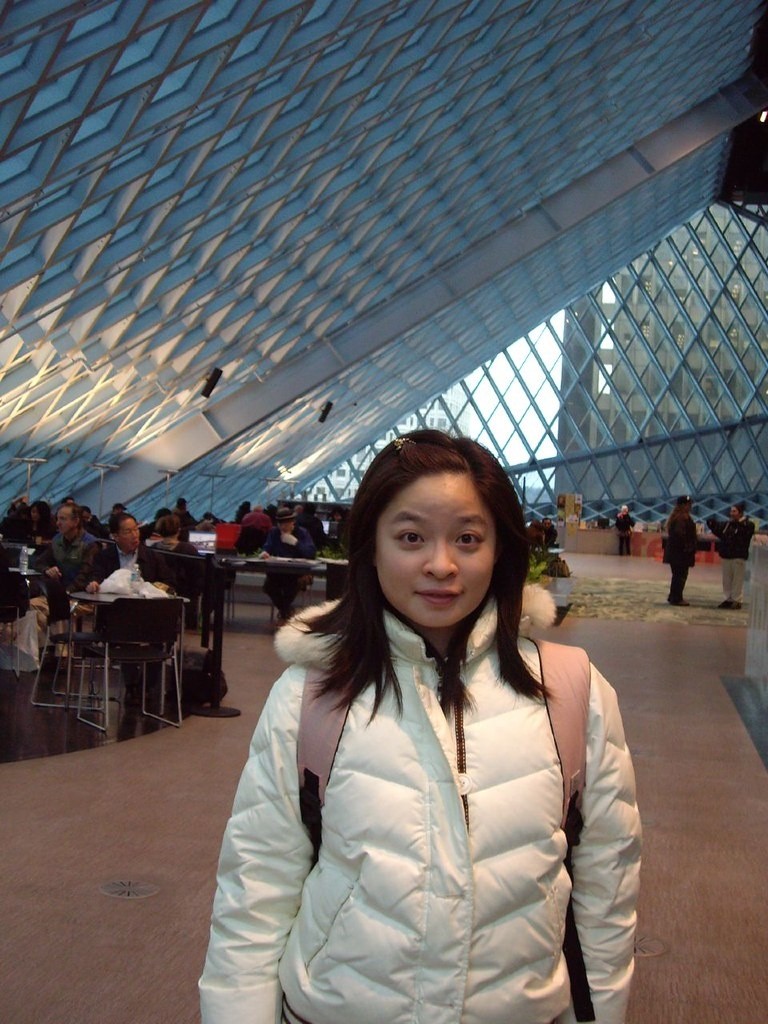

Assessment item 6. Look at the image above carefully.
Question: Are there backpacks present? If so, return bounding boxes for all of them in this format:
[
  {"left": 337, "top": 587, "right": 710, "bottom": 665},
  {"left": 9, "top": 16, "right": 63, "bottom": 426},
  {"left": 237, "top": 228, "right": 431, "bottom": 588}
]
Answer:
[{"left": 170, "top": 644, "right": 227, "bottom": 704}]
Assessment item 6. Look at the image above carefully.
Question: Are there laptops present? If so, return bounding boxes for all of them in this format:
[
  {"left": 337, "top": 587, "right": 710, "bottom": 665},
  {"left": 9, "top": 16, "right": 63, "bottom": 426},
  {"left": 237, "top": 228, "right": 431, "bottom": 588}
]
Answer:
[
  {"left": 188, "top": 531, "right": 217, "bottom": 558},
  {"left": 321, "top": 520, "right": 330, "bottom": 535}
]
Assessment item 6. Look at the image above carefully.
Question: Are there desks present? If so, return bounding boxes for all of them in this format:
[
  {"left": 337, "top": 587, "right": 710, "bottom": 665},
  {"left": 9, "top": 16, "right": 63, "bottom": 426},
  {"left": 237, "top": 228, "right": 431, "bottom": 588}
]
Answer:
[
  {"left": 224, "top": 556, "right": 318, "bottom": 621},
  {"left": 9, "top": 567, "right": 45, "bottom": 576},
  {"left": 558, "top": 529, "right": 620, "bottom": 554},
  {"left": 68, "top": 591, "right": 189, "bottom": 716},
  {"left": 531, "top": 546, "right": 565, "bottom": 576}
]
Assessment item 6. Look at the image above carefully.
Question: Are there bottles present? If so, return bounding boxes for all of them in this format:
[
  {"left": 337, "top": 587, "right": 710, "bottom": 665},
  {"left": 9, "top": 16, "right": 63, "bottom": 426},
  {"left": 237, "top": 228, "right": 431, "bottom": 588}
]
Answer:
[
  {"left": 19, "top": 546, "right": 28, "bottom": 571},
  {"left": 130, "top": 564, "right": 140, "bottom": 594}
]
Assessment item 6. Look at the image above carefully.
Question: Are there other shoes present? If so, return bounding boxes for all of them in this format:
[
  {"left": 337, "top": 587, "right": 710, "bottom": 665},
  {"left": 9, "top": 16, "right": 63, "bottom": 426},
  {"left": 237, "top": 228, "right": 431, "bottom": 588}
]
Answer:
[
  {"left": 730, "top": 602, "right": 741, "bottom": 608},
  {"left": 719, "top": 600, "right": 731, "bottom": 609},
  {"left": 676, "top": 599, "right": 690, "bottom": 606}
]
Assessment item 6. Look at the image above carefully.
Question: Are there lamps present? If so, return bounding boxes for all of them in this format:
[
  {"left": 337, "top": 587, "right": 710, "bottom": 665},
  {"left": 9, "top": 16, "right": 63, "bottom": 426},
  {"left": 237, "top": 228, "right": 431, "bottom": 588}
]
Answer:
[
  {"left": 317, "top": 401, "right": 333, "bottom": 423},
  {"left": 200, "top": 367, "right": 224, "bottom": 398}
]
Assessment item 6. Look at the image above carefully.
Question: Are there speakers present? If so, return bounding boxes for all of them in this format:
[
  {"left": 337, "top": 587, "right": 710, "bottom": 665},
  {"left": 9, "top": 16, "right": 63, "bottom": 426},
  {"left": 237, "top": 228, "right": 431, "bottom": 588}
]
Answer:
[
  {"left": 201, "top": 367, "right": 223, "bottom": 398},
  {"left": 318, "top": 401, "right": 333, "bottom": 423}
]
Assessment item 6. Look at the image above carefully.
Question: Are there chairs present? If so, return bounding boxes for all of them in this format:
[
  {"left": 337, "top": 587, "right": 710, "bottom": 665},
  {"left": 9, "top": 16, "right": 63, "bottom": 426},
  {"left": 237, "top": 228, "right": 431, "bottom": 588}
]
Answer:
[
  {"left": 28, "top": 580, "right": 123, "bottom": 711},
  {"left": 74, "top": 598, "right": 184, "bottom": 731},
  {"left": 0, "top": 571, "right": 30, "bottom": 679}
]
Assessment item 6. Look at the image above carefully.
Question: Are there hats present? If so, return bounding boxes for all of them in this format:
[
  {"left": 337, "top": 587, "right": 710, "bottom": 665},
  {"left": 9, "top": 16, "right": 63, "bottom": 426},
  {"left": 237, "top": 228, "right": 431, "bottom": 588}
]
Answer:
[
  {"left": 274, "top": 508, "right": 296, "bottom": 520},
  {"left": 677, "top": 495, "right": 692, "bottom": 504},
  {"left": 114, "top": 503, "right": 127, "bottom": 510},
  {"left": 734, "top": 500, "right": 746, "bottom": 512}
]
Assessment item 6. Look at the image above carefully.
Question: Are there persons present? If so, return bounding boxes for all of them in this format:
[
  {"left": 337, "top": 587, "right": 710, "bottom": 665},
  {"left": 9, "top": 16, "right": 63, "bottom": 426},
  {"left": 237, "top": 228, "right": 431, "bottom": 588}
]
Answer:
[
  {"left": 197, "top": 427, "right": 642, "bottom": 1023},
  {"left": 1, "top": 491, "right": 754, "bottom": 711}
]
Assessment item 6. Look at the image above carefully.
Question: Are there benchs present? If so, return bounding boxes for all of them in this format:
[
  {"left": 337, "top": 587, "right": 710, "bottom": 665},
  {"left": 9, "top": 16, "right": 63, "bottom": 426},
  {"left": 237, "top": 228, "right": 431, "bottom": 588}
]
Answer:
[{"left": 99, "top": 538, "right": 207, "bottom": 631}]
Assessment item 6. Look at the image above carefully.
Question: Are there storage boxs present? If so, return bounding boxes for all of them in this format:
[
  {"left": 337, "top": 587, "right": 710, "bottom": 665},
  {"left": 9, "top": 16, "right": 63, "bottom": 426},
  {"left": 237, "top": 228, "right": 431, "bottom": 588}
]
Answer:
[{"left": 631, "top": 532, "right": 720, "bottom": 565}]
